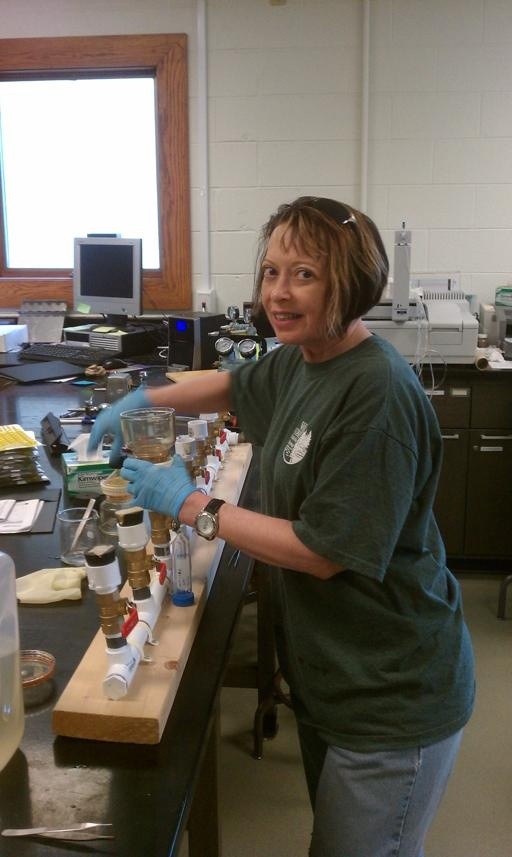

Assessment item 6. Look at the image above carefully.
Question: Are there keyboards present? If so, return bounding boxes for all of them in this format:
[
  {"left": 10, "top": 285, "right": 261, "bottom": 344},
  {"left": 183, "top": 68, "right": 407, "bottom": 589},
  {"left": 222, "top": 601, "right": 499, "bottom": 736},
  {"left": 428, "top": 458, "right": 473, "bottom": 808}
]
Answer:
[{"left": 19, "top": 341, "right": 120, "bottom": 368}]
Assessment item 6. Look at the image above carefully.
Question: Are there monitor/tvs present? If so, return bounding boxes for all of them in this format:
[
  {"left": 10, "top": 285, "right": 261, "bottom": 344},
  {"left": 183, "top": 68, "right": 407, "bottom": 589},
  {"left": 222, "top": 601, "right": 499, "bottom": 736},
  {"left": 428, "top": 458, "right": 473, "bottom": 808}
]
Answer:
[{"left": 72, "top": 239, "right": 143, "bottom": 321}]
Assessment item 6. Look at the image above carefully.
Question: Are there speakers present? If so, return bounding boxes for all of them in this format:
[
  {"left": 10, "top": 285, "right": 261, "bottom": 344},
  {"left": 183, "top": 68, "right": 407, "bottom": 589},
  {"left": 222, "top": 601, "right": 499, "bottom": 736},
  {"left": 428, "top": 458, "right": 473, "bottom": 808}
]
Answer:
[{"left": 164, "top": 308, "right": 224, "bottom": 373}]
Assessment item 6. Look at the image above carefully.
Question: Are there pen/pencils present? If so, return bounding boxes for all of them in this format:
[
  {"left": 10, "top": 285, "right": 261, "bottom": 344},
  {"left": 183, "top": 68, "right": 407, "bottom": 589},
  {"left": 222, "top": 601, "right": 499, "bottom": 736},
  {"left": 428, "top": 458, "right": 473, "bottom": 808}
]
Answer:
[{"left": 60, "top": 410, "right": 80, "bottom": 417}]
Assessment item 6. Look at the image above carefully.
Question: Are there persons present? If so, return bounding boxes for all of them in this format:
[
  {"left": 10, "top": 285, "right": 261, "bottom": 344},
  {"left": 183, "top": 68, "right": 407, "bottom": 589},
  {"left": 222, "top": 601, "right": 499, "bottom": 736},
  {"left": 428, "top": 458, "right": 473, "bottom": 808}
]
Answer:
[{"left": 88, "top": 195, "right": 481, "bottom": 856}]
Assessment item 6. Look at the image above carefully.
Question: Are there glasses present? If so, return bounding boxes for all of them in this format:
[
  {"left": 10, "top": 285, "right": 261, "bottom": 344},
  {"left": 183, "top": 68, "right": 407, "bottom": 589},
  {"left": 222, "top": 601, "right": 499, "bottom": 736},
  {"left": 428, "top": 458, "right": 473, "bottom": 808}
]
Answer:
[{"left": 316, "top": 198, "right": 357, "bottom": 231}]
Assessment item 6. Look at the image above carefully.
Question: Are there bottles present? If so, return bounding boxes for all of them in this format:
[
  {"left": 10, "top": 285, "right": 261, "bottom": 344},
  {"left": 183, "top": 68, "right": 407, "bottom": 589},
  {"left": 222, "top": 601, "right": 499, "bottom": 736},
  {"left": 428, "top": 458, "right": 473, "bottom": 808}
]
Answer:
[
  {"left": 478, "top": 333, "right": 489, "bottom": 348},
  {"left": 171, "top": 533, "right": 195, "bottom": 607},
  {"left": 97, "top": 478, "right": 133, "bottom": 537}
]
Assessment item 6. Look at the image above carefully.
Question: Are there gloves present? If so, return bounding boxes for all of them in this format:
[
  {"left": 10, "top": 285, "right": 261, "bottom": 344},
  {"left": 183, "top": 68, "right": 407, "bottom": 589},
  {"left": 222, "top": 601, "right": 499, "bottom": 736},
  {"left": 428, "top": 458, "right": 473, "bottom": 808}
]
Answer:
[
  {"left": 87, "top": 384, "right": 151, "bottom": 461},
  {"left": 120, "top": 454, "right": 207, "bottom": 525},
  {"left": 15, "top": 565, "right": 86, "bottom": 605}
]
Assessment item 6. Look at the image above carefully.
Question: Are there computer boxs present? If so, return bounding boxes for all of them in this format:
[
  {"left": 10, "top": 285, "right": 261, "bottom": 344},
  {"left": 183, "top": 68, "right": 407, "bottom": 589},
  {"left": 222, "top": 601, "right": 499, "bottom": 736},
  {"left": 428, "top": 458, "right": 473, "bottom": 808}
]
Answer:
[{"left": 61, "top": 323, "right": 165, "bottom": 360}]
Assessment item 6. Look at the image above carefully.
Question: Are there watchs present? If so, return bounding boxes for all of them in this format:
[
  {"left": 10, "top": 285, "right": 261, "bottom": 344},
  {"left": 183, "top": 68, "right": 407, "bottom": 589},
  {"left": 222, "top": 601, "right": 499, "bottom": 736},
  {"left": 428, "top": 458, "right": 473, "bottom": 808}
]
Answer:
[{"left": 194, "top": 497, "right": 226, "bottom": 540}]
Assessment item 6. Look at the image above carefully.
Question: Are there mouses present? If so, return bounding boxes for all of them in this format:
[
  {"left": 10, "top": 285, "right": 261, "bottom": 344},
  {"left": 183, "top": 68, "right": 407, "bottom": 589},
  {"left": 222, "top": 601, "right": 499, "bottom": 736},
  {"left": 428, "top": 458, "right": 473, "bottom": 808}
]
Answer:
[{"left": 105, "top": 358, "right": 128, "bottom": 372}]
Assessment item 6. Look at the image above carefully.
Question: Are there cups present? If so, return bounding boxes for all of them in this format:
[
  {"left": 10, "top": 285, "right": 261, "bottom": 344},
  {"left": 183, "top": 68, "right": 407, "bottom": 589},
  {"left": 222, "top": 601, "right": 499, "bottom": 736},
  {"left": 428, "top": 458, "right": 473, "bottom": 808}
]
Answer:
[{"left": 55, "top": 508, "right": 101, "bottom": 567}]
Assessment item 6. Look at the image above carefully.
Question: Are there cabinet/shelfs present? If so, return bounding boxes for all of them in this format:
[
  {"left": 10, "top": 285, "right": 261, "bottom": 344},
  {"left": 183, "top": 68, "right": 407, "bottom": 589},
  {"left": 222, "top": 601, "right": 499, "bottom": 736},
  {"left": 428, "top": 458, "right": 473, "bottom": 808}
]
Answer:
[{"left": 412, "top": 365, "right": 512, "bottom": 560}]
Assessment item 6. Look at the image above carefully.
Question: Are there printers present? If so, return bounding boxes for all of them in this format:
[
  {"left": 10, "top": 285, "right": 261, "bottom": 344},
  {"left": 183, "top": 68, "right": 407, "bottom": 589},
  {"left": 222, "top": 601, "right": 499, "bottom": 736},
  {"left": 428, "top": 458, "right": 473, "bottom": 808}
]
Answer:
[{"left": 356, "top": 228, "right": 479, "bottom": 365}]
